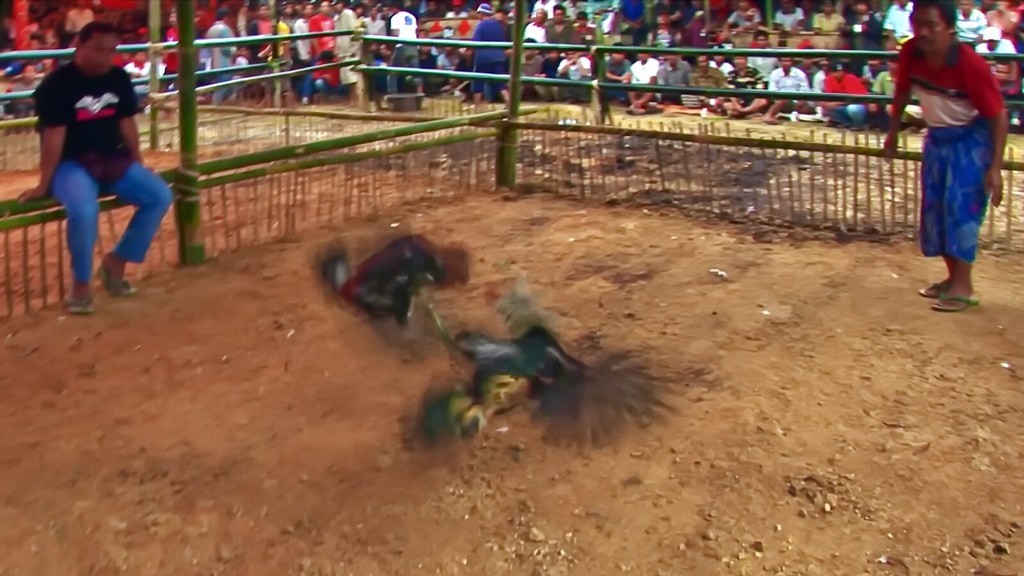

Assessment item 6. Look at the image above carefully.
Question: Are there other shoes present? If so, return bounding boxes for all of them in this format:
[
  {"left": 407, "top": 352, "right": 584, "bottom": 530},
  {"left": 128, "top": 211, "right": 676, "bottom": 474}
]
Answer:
[
  {"left": 70, "top": 293, "right": 95, "bottom": 314},
  {"left": 99, "top": 263, "right": 138, "bottom": 296}
]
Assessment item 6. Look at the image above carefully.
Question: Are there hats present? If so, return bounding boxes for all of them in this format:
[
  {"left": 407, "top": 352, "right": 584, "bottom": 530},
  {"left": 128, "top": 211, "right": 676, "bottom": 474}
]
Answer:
[
  {"left": 452, "top": 0, "right": 463, "bottom": 7},
  {"left": 983, "top": 27, "right": 1002, "bottom": 41},
  {"left": 477, "top": 3, "right": 494, "bottom": 15}
]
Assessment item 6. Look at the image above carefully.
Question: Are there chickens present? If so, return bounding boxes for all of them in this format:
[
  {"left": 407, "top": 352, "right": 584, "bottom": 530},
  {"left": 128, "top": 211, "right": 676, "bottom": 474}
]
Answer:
[
  {"left": 409, "top": 269, "right": 688, "bottom": 451},
  {"left": 319, "top": 234, "right": 471, "bottom": 330}
]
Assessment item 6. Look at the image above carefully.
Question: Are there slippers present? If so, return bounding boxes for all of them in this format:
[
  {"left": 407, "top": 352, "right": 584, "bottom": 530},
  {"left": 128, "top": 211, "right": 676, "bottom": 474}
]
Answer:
[
  {"left": 930, "top": 292, "right": 980, "bottom": 312},
  {"left": 919, "top": 280, "right": 952, "bottom": 299}
]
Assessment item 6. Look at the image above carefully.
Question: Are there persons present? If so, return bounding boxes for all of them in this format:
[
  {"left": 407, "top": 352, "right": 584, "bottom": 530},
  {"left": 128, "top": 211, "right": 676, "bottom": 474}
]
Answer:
[
  {"left": 16, "top": 22, "right": 175, "bottom": 313},
  {"left": 880, "top": 2, "right": 1009, "bottom": 312},
  {"left": 0, "top": 0, "right": 1024, "bottom": 128}
]
